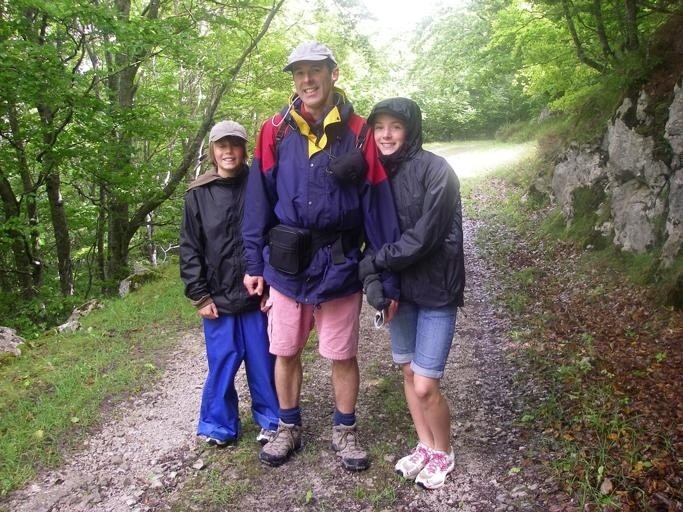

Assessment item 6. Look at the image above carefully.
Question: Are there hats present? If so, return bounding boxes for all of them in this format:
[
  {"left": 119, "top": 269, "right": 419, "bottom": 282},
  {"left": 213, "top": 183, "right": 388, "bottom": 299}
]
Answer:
[
  {"left": 283, "top": 41, "right": 337, "bottom": 74},
  {"left": 209, "top": 121, "right": 249, "bottom": 145}
]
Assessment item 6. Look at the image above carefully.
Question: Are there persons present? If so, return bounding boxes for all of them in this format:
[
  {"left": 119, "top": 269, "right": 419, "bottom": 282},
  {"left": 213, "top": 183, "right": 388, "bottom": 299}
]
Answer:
[
  {"left": 242, "top": 39, "right": 402, "bottom": 473},
  {"left": 179, "top": 120, "right": 278, "bottom": 449},
  {"left": 358, "top": 97, "right": 466, "bottom": 490}
]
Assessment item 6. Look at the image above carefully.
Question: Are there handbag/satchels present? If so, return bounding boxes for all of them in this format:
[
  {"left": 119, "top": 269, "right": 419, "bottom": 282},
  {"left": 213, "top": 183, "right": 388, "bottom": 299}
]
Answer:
[{"left": 269, "top": 225, "right": 311, "bottom": 276}]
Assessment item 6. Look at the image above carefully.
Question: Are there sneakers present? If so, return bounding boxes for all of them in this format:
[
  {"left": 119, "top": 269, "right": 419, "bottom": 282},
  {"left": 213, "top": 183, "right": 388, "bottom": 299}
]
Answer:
[
  {"left": 257, "top": 419, "right": 301, "bottom": 466},
  {"left": 394, "top": 441, "right": 455, "bottom": 489},
  {"left": 206, "top": 436, "right": 227, "bottom": 446},
  {"left": 332, "top": 420, "right": 369, "bottom": 469}
]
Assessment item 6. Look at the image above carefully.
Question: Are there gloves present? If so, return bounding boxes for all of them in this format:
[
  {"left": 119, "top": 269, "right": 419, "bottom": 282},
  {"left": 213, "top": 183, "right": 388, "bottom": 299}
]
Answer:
[{"left": 357, "top": 251, "right": 387, "bottom": 310}]
